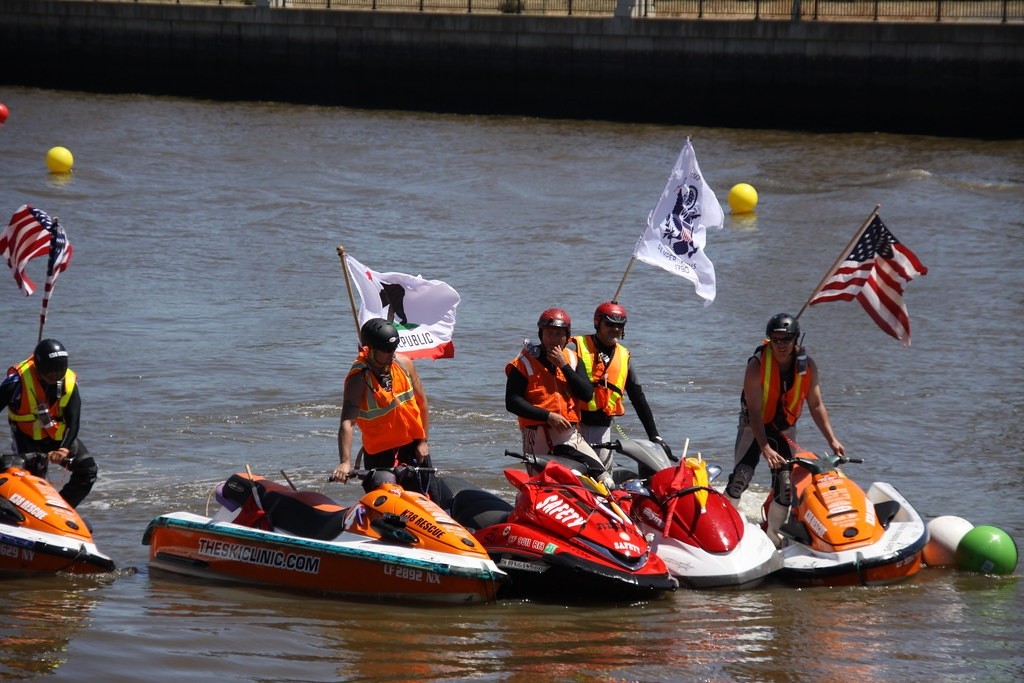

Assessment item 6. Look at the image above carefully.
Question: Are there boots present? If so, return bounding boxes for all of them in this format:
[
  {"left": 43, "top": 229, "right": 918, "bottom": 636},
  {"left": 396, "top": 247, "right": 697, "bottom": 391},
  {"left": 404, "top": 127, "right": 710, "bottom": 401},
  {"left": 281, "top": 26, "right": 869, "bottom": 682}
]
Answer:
[
  {"left": 766, "top": 497, "right": 789, "bottom": 547},
  {"left": 723, "top": 488, "right": 740, "bottom": 510}
]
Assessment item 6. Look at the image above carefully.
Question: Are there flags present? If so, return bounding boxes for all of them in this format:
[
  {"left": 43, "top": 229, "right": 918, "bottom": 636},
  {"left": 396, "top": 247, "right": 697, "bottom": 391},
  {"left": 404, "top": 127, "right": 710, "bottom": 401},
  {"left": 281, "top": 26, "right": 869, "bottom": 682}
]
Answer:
[
  {"left": 804, "top": 212, "right": 928, "bottom": 349},
  {"left": 346, "top": 251, "right": 460, "bottom": 361},
  {"left": 0, "top": 204, "right": 74, "bottom": 325},
  {"left": 631, "top": 136, "right": 726, "bottom": 308}
]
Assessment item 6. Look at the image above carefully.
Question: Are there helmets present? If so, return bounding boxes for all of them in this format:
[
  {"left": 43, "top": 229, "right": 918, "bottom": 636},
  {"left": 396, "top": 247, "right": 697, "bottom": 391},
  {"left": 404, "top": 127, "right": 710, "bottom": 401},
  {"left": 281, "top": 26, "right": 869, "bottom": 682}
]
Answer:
[
  {"left": 33, "top": 339, "right": 68, "bottom": 382},
  {"left": 594, "top": 302, "right": 627, "bottom": 331},
  {"left": 766, "top": 313, "right": 800, "bottom": 338},
  {"left": 538, "top": 309, "right": 571, "bottom": 327},
  {"left": 360, "top": 318, "right": 400, "bottom": 353}
]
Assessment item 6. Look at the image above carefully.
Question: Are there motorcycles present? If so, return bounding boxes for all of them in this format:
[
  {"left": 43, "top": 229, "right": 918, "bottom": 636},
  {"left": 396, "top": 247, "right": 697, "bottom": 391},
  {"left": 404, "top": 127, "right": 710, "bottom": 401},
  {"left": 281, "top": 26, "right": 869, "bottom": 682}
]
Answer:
[
  {"left": 591, "top": 434, "right": 783, "bottom": 591},
  {"left": 141, "top": 463, "right": 507, "bottom": 601},
  {"left": 752, "top": 451, "right": 930, "bottom": 588},
  {"left": 0, "top": 452, "right": 118, "bottom": 574},
  {"left": 437, "top": 450, "right": 679, "bottom": 603}
]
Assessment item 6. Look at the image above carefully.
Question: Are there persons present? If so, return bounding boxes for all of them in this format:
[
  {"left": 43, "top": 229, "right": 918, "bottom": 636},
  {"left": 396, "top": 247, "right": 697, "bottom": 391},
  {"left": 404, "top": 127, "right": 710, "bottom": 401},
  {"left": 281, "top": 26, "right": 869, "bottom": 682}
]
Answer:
[
  {"left": 567, "top": 301, "right": 674, "bottom": 468},
  {"left": 331, "top": 318, "right": 441, "bottom": 510},
  {"left": 720, "top": 314, "right": 846, "bottom": 550},
  {"left": 507, "top": 306, "right": 613, "bottom": 490},
  {"left": 0, "top": 339, "right": 97, "bottom": 510}
]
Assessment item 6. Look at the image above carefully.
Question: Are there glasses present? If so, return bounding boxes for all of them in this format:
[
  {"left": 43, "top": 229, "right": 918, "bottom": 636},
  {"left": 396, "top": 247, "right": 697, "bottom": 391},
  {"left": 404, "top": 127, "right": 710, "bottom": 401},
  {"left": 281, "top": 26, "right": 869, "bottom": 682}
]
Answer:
[
  {"left": 603, "top": 317, "right": 625, "bottom": 328},
  {"left": 769, "top": 335, "right": 796, "bottom": 344}
]
